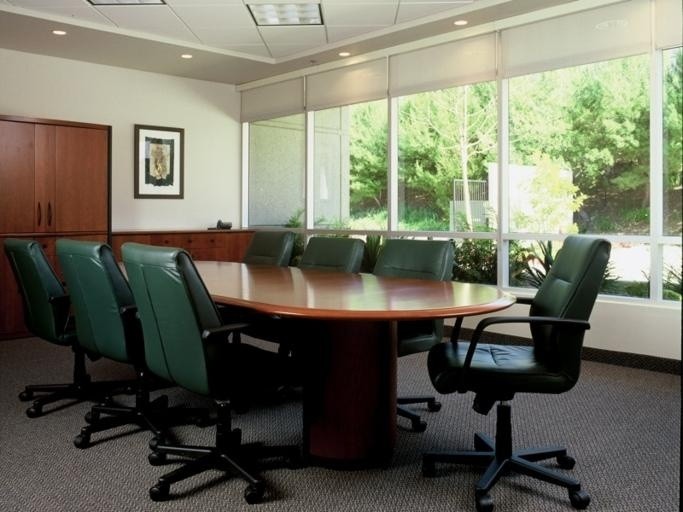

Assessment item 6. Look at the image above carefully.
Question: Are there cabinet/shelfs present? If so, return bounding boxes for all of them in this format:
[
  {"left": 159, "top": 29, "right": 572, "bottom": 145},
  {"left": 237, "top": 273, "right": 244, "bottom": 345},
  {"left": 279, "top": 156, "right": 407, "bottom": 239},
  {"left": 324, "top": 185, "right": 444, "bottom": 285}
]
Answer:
[{"left": 0, "top": 115, "right": 110, "bottom": 342}]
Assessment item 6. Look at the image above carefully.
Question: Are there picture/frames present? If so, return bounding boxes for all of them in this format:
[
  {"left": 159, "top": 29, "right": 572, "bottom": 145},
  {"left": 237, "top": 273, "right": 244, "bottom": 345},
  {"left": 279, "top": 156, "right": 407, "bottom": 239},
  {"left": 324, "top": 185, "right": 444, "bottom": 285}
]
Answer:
[{"left": 133, "top": 123, "right": 185, "bottom": 200}]
